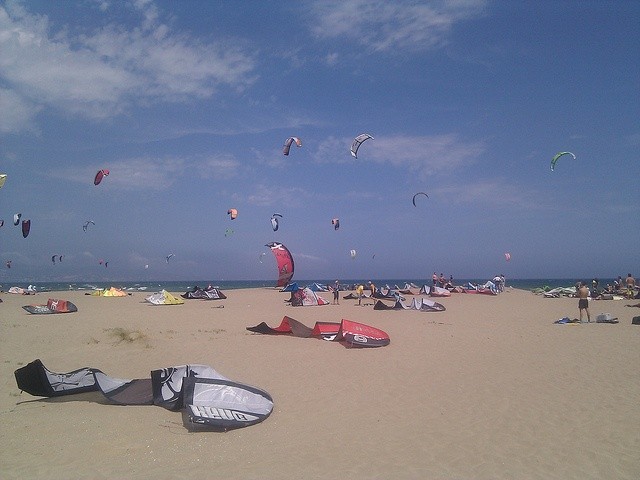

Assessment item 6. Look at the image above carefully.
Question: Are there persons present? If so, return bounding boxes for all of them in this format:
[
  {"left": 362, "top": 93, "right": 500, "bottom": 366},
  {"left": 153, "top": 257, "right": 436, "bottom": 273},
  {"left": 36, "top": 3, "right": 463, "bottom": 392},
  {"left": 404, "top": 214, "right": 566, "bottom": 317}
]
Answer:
[
  {"left": 499, "top": 274, "right": 506, "bottom": 292},
  {"left": 493, "top": 275, "right": 500, "bottom": 292},
  {"left": 626, "top": 274, "right": 636, "bottom": 300},
  {"left": 432, "top": 272, "right": 438, "bottom": 286},
  {"left": 27, "top": 283, "right": 38, "bottom": 294},
  {"left": 613, "top": 280, "right": 618, "bottom": 288},
  {"left": 439, "top": 273, "right": 444, "bottom": 287},
  {"left": 618, "top": 276, "right": 623, "bottom": 287},
  {"left": 332, "top": 281, "right": 341, "bottom": 305},
  {"left": 368, "top": 280, "right": 378, "bottom": 305},
  {"left": 578, "top": 281, "right": 591, "bottom": 322},
  {"left": 355, "top": 283, "right": 365, "bottom": 305}
]
